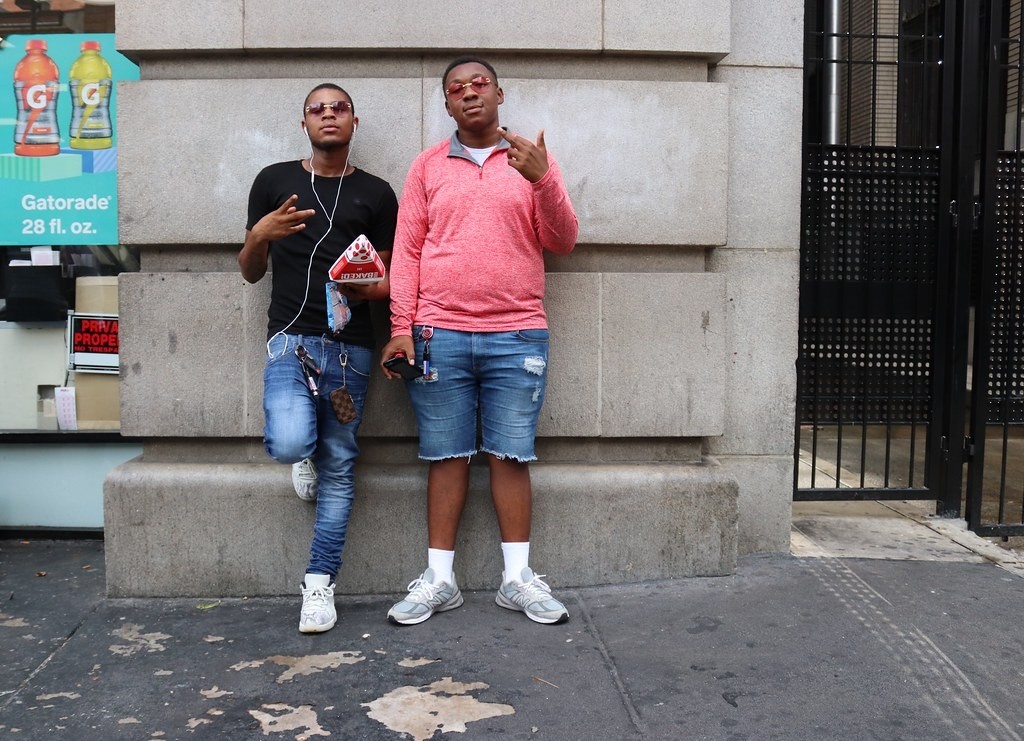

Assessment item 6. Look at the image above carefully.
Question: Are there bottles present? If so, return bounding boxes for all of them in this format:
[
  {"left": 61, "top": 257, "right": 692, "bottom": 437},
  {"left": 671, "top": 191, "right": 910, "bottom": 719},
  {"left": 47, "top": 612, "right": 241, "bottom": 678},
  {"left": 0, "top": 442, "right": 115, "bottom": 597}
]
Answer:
[
  {"left": 13, "top": 40, "right": 60, "bottom": 157},
  {"left": 69, "top": 41, "right": 113, "bottom": 149}
]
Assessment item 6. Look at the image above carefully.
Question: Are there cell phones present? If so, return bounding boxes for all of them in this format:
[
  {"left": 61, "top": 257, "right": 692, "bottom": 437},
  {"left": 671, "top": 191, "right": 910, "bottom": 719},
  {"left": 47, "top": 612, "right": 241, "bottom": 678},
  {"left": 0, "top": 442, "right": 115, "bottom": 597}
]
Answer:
[{"left": 384, "top": 355, "right": 424, "bottom": 381}]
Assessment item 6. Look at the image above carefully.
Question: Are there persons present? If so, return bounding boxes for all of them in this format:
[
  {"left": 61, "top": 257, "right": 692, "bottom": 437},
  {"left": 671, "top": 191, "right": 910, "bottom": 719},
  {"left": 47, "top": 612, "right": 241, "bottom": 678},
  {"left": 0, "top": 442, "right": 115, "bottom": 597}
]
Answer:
[
  {"left": 382, "top": 56, "right": 580, "bottom": 626},
  {"left": 239, "top": 84, "right": 399, "bottom": 633}
]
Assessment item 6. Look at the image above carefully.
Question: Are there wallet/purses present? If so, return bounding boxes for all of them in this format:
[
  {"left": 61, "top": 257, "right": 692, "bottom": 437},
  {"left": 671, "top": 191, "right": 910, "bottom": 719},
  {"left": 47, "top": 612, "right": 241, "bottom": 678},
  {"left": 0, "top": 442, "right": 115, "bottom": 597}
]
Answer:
[{"left": 329, "top": 385, "right": 359, "bottom": 424}]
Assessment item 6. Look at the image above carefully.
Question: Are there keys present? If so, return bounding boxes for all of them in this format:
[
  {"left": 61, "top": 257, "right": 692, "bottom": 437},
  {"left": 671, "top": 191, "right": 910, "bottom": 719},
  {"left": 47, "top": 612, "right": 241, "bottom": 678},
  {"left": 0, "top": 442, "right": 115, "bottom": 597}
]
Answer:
[
  {"left": 423, "top": 345, "right": 430, "bottom": 379},
  {"left": 300, "top": 355, "right": 322, "bottom": 396}
]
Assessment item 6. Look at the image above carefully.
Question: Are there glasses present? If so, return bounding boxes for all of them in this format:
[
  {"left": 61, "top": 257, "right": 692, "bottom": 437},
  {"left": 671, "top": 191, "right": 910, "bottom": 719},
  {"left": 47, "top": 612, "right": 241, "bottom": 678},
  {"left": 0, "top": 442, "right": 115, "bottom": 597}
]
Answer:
[
  {"left": 306, "top": 101, "right": 354, "bottom": 117},
  {"left": 445, "top": 76, "right": 498, "bottom": 101}
]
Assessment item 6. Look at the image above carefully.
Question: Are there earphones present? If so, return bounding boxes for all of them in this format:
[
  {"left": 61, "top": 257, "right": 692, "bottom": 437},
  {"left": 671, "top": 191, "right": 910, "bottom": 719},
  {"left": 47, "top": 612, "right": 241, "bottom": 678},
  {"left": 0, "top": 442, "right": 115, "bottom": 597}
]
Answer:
[
  {"left": 354, "top": 123, "right": 357, "bottom": 132},
  {"left": 304, "top": 126, "right": 308, "bottom": 135}
]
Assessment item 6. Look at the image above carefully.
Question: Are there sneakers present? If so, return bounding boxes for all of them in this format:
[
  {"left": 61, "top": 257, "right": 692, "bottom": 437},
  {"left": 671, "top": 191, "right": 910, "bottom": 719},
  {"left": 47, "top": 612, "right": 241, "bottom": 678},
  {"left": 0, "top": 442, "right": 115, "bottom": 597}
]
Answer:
[
  {"left": 495, "top": 566, "right": 571, "bottom": 625},
  {"left": 292, "top": 458, "right": 319, "bottom": 502},
  {"left": 386, "top": 567, "right": 465, "bottom": 624},
  {"left": 300, "top": 574, "right": 338, "bottom": 631}
]
